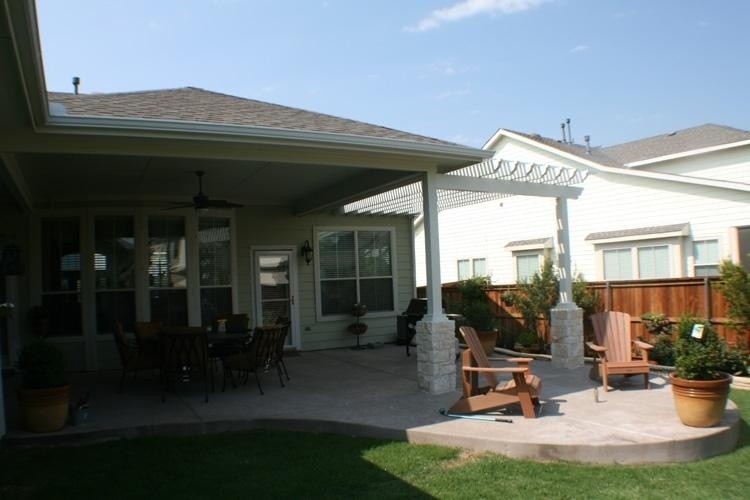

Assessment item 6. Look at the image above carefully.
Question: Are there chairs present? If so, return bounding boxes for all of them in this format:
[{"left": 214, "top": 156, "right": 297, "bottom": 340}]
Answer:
[
  {"left": 585, "top": 311, "right": 654, "bottom": 393},
  {"left": 447, "top": 326, "right": 545, "bottom": 418},
  {"left": 111, "top": 312, "right": 292, "bottom": 403}
]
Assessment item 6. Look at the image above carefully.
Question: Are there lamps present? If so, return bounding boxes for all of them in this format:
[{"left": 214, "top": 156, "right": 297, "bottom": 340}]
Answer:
[{"left": 300, "top": 238, "right": 314, "bottom": 265}]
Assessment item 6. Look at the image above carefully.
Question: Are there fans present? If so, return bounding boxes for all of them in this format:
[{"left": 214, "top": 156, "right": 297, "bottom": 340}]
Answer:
[{"left": 158, "top": 170, "right": 245, "bottom": 212}]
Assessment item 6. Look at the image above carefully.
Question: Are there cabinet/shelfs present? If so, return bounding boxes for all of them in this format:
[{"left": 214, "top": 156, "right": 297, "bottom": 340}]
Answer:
[{"left": 397, "top": 314, "right": 462, "bottom": 357}]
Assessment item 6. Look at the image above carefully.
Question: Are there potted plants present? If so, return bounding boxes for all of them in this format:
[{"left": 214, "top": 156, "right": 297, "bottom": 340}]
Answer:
[
  {"left": 672, "top": 312, "right": 742, "bottom": 428},
  {"left": 458, "top": 298, "right": 502, "bottom": 356},
  {"left": 12, "top": 339, "right": 75, "bottom": 434},
  {"left": 513, "top": 331, "right": 541, "bottom": 353}
]
